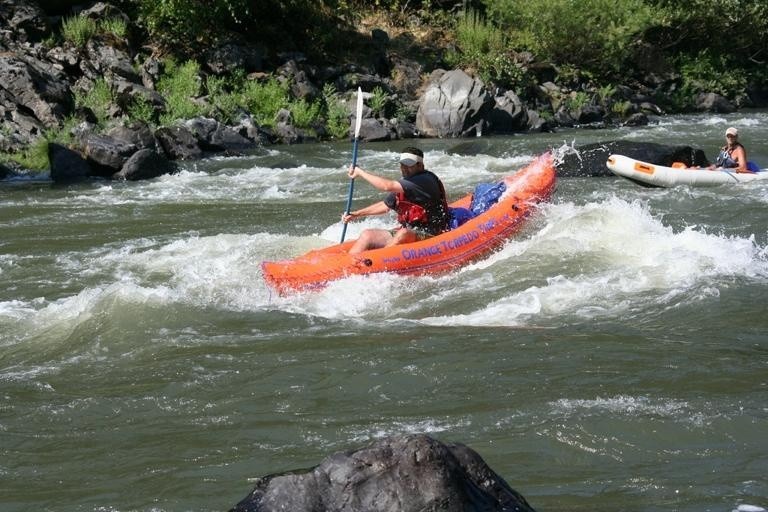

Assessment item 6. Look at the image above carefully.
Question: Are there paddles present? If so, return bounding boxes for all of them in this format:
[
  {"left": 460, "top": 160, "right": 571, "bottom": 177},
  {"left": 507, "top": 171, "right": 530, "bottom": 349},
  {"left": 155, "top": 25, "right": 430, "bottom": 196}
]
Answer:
[
  {"left": 340, "top": 86, "right": 363, "bottom": 246},
  {"left": 717, "top": 168, "right": 756, "bottom": 174}
]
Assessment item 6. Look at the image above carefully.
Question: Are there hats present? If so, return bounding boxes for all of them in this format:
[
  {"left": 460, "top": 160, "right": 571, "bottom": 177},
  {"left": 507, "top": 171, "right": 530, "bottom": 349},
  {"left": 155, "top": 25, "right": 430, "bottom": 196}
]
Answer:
[
  {"left": 392, "top": 153, "right": 423, "bottom": 167},
  {"left": 726, "top": 128, "right": 738, "bottom": 136}
]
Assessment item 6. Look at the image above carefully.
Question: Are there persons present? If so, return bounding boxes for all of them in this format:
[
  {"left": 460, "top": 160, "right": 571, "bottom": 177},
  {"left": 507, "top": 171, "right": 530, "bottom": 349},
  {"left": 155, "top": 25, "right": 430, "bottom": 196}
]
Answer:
[
  {"left": 689, "top": 128, "right": 747, "bottom": 172},
  {"left": 342, "top": 146, "right": 449, "bottom": 253}
]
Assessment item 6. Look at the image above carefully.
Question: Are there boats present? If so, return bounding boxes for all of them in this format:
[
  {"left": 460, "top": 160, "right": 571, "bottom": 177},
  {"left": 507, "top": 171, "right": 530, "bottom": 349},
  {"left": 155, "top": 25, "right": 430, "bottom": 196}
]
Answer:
[
  {"left": 605, "top": 154, "right": 768, "bottom": 190},
  {"left": 261, "top": 149, "right": 557, "bottom": 300}
]
